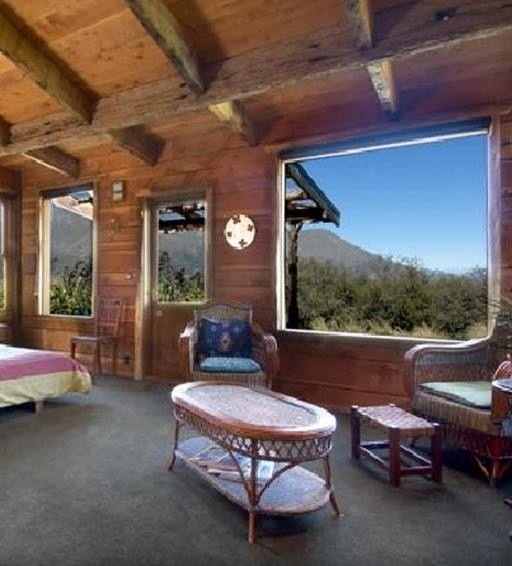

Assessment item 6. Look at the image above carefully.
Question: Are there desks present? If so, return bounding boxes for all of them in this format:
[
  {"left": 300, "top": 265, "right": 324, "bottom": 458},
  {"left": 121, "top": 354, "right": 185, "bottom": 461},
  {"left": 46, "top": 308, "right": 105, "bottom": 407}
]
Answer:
[{"left": 165, "top": 381, "right": 344, "bottom": 544}]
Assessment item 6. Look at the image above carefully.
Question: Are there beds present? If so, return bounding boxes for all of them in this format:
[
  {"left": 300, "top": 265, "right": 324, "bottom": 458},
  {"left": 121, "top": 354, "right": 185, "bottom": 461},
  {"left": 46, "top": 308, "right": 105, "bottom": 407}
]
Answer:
[{"left": 0, "top": 342, "right": 92, "bottom": 416}]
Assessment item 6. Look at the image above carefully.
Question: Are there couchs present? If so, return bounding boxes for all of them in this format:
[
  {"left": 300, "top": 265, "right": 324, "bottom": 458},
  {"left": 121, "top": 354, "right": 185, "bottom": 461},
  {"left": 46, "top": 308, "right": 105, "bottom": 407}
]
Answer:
[{"left": 402, "top": 321, "right": 512, "bottom": 489}]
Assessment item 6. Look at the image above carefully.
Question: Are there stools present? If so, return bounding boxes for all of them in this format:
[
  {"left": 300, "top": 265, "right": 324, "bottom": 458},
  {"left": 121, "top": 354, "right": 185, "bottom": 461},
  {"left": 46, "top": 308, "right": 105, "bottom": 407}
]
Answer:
[{"left": 349, "top": 401, "right": 446, "bottom": 486}]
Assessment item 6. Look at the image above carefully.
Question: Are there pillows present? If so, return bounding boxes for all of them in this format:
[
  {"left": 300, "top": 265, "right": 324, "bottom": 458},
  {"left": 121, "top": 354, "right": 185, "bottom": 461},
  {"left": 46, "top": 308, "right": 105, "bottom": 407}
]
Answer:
[
  {"left": 492, "top": 359, "right": 512, "bottom": 381},
  {"left": 197, "top": 315, "right": 252, "bottom": 354}
]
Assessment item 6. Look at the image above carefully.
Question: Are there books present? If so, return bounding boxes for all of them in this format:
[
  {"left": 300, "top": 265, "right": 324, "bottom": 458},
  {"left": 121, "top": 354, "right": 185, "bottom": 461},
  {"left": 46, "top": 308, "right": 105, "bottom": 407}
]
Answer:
[
  {"left": 206, "top": 459, "right": 252, "bottom": 476},
  {"left": 219, "top": 473, "right": 269, "bottom": 486},
  {"left": 189, "top": 442, "right": 231, "bottom": 465},
  {"left": 217, "top": 458, "right": 275, "bottom": 481},
  {"left": 206, "top": 455, "right": 249, "bottom": 470}
]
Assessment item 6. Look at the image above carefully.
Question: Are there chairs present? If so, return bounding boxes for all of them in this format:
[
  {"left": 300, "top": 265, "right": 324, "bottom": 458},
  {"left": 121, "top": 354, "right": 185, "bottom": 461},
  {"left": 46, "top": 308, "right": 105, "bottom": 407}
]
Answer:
[
  {"left": 71, "top": 295, "right": 124, "bottom": 379},
  {"left": 177, "top": 304, "right": 280, "bottom": 387}
]
